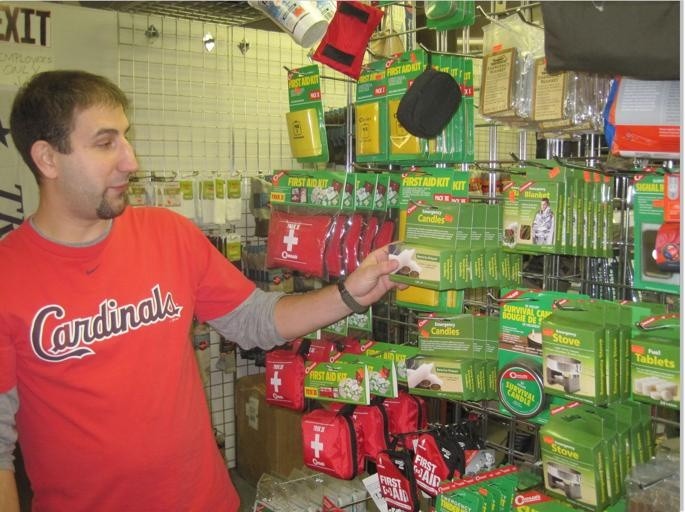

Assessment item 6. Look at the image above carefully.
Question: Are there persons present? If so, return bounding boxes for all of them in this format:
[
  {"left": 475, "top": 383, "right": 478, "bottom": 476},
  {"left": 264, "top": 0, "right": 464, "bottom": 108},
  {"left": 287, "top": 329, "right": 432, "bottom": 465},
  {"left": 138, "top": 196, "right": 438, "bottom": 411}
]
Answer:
[{"left": 1, "top": 71, "right": 414, "bottom": 512}]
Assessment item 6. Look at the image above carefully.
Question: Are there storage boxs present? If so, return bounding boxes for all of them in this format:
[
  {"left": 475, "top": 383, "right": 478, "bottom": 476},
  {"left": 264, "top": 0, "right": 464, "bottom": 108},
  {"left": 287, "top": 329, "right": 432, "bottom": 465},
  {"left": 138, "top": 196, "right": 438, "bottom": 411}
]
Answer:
[{"left": 235, "top": 371, "right": 321, "bottom": 488}]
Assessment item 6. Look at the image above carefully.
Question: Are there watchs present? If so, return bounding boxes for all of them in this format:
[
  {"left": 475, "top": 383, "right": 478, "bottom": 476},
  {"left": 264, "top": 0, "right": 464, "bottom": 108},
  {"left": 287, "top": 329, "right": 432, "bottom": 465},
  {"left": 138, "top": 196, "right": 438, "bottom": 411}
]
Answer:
[{"left": 334, "top": 275, "right": 369, "bottom": 317}]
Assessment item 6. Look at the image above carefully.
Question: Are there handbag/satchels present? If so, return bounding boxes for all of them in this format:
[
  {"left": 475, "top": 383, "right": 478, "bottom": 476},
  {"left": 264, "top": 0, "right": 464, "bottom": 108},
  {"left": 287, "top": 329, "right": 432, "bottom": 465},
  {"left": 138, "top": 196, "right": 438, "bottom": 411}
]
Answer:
[{"left": 396, "top": 51, "right": 463, "bottom": 140}]
